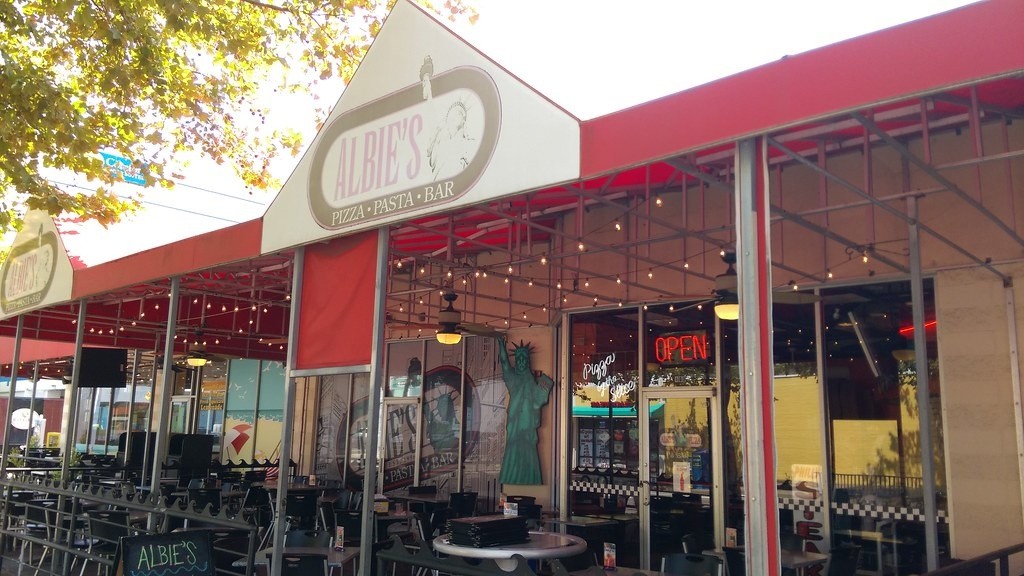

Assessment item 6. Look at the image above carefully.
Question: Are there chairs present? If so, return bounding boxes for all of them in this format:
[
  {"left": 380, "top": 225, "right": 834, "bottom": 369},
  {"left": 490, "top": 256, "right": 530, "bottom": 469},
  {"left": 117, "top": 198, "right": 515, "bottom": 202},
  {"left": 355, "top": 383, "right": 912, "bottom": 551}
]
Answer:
[{"left": 0, "top": 446, "right": 827, "bottom": 576}]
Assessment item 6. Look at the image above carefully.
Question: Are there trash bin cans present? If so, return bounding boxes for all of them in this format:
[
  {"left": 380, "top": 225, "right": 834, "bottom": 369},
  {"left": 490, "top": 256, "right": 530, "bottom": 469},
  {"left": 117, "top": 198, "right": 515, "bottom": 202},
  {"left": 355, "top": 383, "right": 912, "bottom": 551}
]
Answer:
[{"left": 22, "top": 447, "right": 61, "bottom": 483}]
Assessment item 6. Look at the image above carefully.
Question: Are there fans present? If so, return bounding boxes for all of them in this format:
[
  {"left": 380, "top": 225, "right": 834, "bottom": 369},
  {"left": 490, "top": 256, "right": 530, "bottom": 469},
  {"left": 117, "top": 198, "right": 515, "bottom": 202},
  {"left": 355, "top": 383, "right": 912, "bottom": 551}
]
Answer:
[{"left": 140, "top": 355, "right": 195, "bottom": 373}]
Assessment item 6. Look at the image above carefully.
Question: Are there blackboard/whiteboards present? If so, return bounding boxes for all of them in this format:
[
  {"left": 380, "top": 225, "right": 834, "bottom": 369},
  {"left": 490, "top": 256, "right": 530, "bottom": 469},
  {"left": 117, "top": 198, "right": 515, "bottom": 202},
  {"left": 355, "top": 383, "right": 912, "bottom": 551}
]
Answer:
[{"left": 121, "top": 529, "right": 216, "bottom": 576}]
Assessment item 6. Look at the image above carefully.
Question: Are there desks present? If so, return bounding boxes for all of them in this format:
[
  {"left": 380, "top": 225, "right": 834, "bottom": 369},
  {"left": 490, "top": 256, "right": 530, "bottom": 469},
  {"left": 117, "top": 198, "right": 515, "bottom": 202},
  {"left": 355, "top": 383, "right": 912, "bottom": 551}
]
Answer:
[
  {"left": 702, "top": 545, "right": 827, "bottom": 576},
  {"left": 232, "top": 545, "right": 360, "bottom": 576},
  {"left": 261, "top": 483, "right": 330, "bottom": 537},
  {"left": 537, "top": 516, "right": 615, "bottom": 567},
  {"left": 433, "top": 531, "right": 586, "bottom": 559},
  {"left": 398, "top": 493, "right": 450, "bottom": 524}
]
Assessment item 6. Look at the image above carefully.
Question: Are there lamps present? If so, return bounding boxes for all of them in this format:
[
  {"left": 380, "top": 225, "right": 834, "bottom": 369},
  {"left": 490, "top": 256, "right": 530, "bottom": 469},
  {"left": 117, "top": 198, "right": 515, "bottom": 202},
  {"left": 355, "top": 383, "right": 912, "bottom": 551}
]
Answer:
[
  {"left": 435, "top": 293, "right": 462, "bottom": 346},
  {"left": 715, "top": 249, "right": 740, "bottom": 319},
  {"left": 186, "top": 331, "right": 208, "bottom": 366},
  {"left": 63, "top": 360, "right": 74, "bottom": 380}
]
